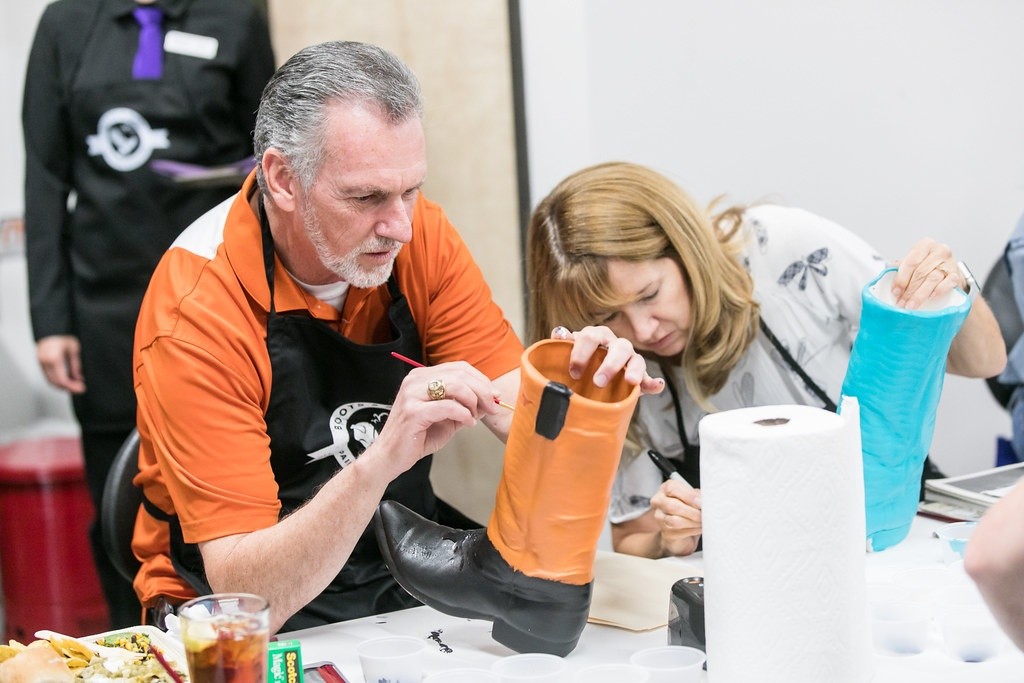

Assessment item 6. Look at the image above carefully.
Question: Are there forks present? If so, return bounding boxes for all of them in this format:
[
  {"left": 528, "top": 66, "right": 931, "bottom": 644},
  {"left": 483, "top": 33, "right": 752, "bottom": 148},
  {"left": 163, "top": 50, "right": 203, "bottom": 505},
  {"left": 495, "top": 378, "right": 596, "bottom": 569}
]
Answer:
[{"left": 34, "top": 631, "right": 147, "bottom": 662}]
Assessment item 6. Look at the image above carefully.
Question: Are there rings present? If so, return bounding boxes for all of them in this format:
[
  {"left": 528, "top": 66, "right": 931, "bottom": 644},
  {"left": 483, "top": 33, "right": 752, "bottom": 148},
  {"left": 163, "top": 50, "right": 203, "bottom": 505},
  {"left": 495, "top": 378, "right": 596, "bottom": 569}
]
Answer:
[
  {"left": 935, "top": 265, "right": 950, "bottom": 277},
  {"left": 427, "top": 380, "right": 446, "bottom": 400}
]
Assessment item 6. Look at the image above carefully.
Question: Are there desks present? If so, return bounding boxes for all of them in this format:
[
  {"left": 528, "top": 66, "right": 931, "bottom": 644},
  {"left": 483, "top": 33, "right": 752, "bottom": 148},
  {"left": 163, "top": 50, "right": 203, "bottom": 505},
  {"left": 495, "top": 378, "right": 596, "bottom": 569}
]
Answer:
[{"left": 278, "top": 513, "right": 949, "bottom": 683}]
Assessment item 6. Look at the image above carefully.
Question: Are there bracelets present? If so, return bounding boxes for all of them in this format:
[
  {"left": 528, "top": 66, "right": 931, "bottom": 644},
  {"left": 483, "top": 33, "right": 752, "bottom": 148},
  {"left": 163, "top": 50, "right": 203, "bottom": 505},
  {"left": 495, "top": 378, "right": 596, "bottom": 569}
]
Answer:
[{"left": 957, "top": 264, "right": 979, "bottom": 304}]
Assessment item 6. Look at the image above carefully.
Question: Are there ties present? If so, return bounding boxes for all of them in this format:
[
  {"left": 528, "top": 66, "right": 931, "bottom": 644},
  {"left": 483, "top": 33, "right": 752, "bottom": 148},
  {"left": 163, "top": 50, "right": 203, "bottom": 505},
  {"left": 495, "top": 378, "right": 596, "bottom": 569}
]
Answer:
[{"left": 130, "top": 7, "right": 163, "bottom": 78}]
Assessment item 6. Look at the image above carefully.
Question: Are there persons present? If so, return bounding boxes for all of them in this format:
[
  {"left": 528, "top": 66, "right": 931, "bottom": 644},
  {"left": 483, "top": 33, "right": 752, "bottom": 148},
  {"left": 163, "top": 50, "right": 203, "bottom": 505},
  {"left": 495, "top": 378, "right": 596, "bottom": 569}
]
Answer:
[
  {"left": 977, "top": 211, "right": 1024, "bottom": 462},
  {"left": 534, "top": 162, "right": 1007, "bottom": 558},
  {"left": 126, "top": 40, "right": 665, "bottom": 642},
  {"left": 19, "top": 0, "right": 277, "bottom": 630},
  {"left": 962, "top": 479, "right": 1024, "bottom": 655}
]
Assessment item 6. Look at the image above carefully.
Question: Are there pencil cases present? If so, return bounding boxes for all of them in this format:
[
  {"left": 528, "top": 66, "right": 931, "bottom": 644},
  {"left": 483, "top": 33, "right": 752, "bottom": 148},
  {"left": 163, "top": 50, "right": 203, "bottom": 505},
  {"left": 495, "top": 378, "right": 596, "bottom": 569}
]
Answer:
[{"left": 302, "top": 659, "right": 349, "bottom": 683}]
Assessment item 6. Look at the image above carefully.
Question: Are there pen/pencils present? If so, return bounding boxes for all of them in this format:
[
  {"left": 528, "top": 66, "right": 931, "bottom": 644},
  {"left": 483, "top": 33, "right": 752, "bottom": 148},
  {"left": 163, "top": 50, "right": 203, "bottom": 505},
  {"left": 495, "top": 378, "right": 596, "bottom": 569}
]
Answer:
[
  {"left": 648, "top": 448, "right": 694, "bottom": 490},
  {"left": 146, "top": 642, "right": 184, "bottom": 682}
]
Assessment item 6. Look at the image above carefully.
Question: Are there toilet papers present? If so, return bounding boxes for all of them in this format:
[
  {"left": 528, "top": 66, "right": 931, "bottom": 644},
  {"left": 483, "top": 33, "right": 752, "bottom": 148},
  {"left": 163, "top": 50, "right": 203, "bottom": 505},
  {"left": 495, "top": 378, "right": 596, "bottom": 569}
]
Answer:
[{"left": 699, "top": 395, "right": 865, "bottom": 682}]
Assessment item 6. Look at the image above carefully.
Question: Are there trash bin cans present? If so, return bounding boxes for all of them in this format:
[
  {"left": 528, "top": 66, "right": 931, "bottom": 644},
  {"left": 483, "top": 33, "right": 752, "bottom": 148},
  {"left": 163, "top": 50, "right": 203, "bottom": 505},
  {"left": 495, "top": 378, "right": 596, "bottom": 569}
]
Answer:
[{"left": 0, "top": 437, "right": 109, "bottom": 646}]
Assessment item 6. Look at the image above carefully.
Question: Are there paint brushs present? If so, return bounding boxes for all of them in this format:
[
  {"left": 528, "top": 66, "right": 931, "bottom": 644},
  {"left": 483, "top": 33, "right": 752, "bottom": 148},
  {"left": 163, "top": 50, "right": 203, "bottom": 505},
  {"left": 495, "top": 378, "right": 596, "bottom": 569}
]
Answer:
[
  {"left": 318, "top": 665, "right": 346, "bottom": 683},
  {"left": 393, "top": 352, "right": 516, "bottom": 412}
]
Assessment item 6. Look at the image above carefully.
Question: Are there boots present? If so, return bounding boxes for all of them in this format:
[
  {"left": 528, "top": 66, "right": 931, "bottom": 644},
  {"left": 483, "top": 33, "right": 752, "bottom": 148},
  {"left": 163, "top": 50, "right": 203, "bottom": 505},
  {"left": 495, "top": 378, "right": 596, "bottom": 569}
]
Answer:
[{"left": 373, "top": 339, "right": 641, "bottom": 658}]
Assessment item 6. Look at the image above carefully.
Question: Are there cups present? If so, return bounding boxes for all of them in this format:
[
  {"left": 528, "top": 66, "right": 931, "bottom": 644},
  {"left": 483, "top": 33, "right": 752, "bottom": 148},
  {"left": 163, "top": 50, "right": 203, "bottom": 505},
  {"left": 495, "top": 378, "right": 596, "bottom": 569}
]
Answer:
[
  {"left": 631, "top": 646, "right": 706, "bottom": 683},
  {"left": 357, "top": 636, "right": 428, "bottom": 683},
  {"left": 424, "top": 669, "right": 499, "bottom": 683},
  {"left": 491, "top": 653, "right": 566, "bottom": 683},
  {"left": 569, "top": 664, "right": 650, "bottom": 683},
  {"left": 178, "top": 593, "right": 270, "bottom": 683}
]
